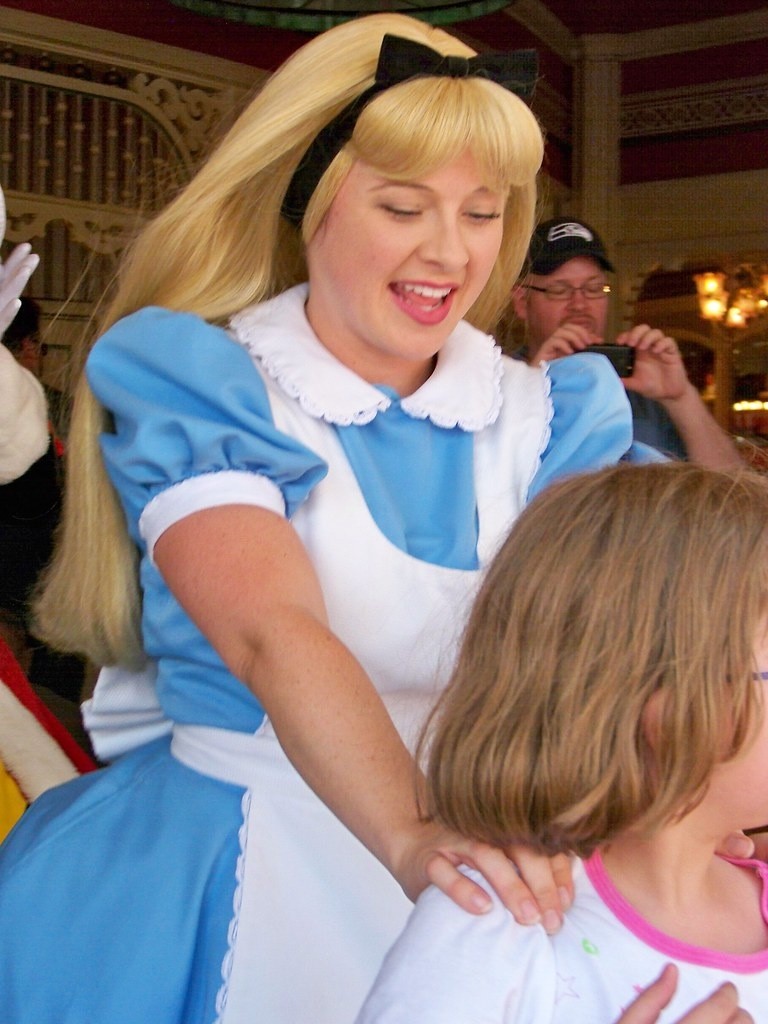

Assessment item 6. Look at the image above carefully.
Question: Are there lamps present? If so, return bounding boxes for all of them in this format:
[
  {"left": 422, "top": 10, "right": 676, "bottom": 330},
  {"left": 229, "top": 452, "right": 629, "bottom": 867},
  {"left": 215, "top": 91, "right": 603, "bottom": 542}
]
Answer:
[{"left": 693, "top": 269, "right": 768, "bottom": 327}]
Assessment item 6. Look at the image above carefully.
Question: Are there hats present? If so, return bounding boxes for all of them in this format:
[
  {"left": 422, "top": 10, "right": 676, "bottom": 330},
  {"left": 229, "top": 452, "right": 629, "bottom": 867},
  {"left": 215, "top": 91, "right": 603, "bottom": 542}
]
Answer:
[{"left": 528, "top": 216, "right": 616, "bottom": 275}]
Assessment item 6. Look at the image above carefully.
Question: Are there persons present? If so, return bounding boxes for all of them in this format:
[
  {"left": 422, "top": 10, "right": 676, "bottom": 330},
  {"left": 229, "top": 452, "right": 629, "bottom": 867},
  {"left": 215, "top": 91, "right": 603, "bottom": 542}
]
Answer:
[
  {"left": 0, "top": 184, "right": 100, "bottom": 847},
  {"left": 507, "top": 217, "right": 744, "bottom": 470},
  {"left": 353, "top": 463, "right": 768, "bottom": 1024},
  {"left": 0, "top": 13, "right": 673, "bottom": 1024}
]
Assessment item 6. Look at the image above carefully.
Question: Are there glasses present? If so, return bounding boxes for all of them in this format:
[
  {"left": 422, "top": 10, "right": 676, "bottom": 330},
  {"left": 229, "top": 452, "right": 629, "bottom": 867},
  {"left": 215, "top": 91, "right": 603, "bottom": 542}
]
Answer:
[
  {"left": 31, "top": 338, "right": 49, "bottom": 356},
  {"left": 522, "top": 283, "right": 612, "bottom": 301}
]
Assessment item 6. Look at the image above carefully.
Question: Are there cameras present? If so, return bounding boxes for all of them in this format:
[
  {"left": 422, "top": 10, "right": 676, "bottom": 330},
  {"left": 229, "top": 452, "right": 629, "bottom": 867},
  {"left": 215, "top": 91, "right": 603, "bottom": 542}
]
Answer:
[{"left": 574, "top": 343, "right": 634, "bottom": 376}]
